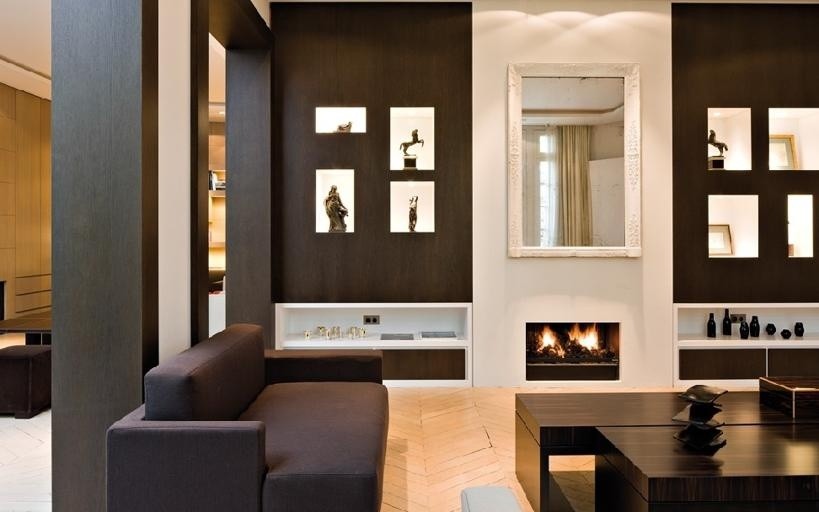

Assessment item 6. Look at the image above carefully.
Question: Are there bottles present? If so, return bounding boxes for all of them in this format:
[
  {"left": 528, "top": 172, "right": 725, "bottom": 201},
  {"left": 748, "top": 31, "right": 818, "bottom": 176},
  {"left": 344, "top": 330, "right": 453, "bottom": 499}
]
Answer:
[
  {"left": 781, "top": 329, "right": 792, "bottom": 339},
  {"left": 765, "top": 323, "right": 776, "bottom": 335},
  {"left": 739, "top": 314, "right": 760, "bottom": 338},
  {"left": 794, "top": 322, "right": 804, "bottom": 337},
  {"left": 706, "top": 311, "right": 717, "bottom": 338},
  {"left": 722, "top": 308, "right": 733, "bottom": 337}
]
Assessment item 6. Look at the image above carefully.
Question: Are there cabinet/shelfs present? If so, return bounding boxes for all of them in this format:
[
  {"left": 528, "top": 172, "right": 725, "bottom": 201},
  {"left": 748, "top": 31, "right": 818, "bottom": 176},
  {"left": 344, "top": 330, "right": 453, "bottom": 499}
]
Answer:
[
  {"left": 276, "top": 302, "right": 471, "bottom": 387},
  {"left": 672, "top": 301, "right": 818, "bottom": 381}
]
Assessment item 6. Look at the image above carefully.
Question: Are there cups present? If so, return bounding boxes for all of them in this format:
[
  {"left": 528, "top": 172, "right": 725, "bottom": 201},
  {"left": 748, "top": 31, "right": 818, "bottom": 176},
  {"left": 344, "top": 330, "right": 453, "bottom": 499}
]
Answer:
[{"left": 304, "top": 326, "right": 367, "bottom": 342}]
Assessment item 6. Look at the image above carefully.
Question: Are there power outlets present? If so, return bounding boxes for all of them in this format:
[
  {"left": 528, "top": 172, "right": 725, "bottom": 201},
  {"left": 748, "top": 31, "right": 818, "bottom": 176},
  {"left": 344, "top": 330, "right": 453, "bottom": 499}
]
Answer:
[
  {"left": 363, "top": 315, "right": 380, "bottom": 325},
  {"left": 730, "top": 314, "right": 746, "bottom": 324}
]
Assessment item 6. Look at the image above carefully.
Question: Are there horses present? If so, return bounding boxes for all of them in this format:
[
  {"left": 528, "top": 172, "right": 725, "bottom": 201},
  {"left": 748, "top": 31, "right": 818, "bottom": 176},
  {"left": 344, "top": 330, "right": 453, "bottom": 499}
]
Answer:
[
  {"left": 707, "top": 129, "right": 727, "bottom": 156},
  {"left": 400, "top": 129, "right": 424, "bottom": 157}
]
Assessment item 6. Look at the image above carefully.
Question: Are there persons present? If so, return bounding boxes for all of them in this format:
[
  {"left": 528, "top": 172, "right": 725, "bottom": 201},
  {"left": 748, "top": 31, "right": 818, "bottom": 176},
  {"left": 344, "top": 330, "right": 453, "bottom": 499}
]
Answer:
[
  {"left": 408, "top": 194, "right": 418, "bottom": 230},
  {"left": 323, "top": 185, "right": 349, "bottom": 233}
]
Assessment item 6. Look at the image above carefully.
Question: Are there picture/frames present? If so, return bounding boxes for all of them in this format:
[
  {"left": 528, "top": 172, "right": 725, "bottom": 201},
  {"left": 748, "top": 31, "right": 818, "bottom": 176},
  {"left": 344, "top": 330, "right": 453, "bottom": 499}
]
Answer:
[
  {"left": 709, "top": 224, "right": 734, "bottom": 256},
  {"left": 768, "top": 133, "right": 798, "bottom": 170}
]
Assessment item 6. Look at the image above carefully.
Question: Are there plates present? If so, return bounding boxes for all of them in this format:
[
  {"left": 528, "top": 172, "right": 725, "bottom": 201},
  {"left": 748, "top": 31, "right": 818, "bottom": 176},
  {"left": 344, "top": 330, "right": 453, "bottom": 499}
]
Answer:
[
  {"left": 678, "top": 384, "right": 729, "bottom": 405},
  {"left": 671, "top": 422, "right": 727, "bottom": 457}
]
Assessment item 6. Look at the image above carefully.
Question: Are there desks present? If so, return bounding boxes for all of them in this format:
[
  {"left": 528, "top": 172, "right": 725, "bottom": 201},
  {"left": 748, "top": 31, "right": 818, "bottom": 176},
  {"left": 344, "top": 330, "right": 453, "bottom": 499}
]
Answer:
[
  {"left": 516, "top": 391, "right": 819, "bottom": 511},
  {"left": 0, "top": 309, "right": 52, "bottom": 345},
  {"left": 595, "top": 424, "right": 819, "bottom": 512}
]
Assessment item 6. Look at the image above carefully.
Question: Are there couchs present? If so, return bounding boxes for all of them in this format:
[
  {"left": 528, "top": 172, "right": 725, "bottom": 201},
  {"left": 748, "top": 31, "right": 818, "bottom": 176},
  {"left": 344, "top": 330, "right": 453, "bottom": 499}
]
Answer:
[{"left": 105, "top": 324, "right": 388, "bottom": 511}]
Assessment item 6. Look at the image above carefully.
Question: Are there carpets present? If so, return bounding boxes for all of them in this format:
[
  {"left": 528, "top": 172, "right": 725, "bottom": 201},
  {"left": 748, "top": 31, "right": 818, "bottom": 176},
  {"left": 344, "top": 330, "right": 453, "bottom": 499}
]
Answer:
[{"left": 461, "top": 487, "right": 521, "bottom": 512}]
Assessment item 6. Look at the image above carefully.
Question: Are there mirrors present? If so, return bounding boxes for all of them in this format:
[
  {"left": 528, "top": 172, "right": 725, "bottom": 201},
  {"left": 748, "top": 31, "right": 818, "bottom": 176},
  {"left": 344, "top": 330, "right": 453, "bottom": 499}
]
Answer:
[{"left": 507, "top": 63, "right": 642, "bottom": 259}]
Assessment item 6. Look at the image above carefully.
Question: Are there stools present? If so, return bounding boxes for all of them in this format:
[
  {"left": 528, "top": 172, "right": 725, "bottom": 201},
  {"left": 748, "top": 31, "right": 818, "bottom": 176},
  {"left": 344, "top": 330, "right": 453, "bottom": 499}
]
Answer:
[{"left": 0, "top": 344, "right": 51, "bottom": 419}]
